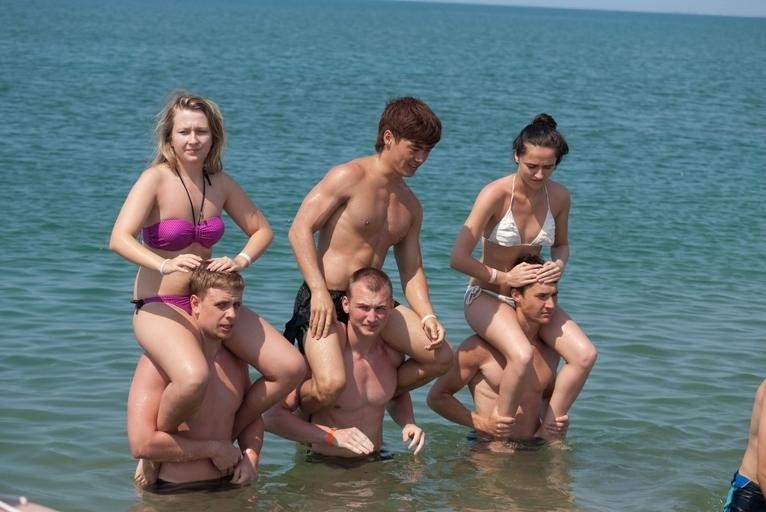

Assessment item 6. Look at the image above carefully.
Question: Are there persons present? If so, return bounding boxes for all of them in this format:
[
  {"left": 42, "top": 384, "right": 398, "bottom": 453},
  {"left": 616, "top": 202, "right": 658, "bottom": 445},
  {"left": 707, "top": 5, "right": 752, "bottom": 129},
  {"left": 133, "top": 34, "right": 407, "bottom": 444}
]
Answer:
[{"left": 724, "top": 378, "right": 766, "bottom": 512}]
